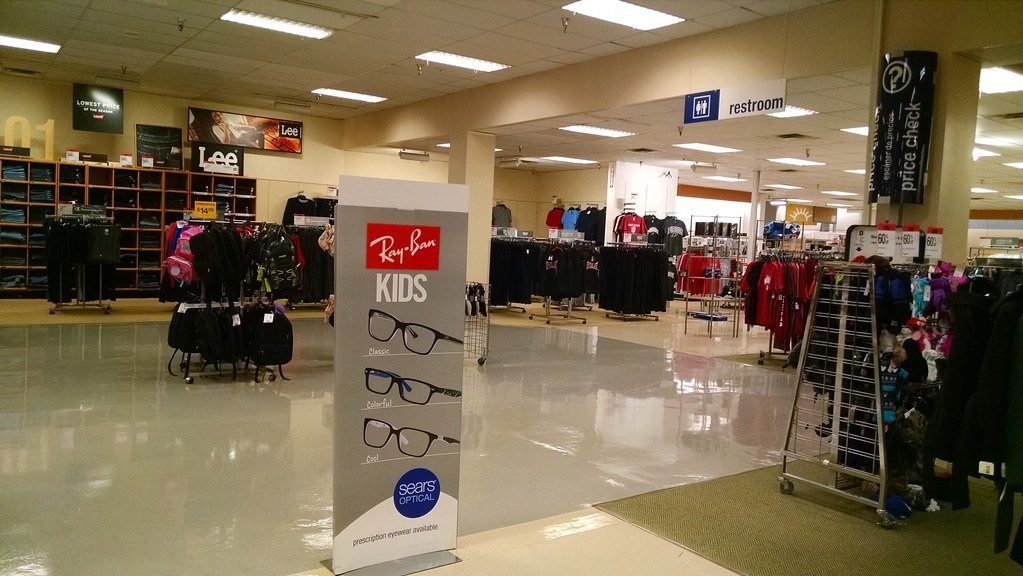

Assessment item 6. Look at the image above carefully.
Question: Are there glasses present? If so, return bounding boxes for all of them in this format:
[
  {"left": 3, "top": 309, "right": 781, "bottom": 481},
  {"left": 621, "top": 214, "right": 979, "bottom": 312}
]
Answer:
[
  {"left": 363, "top": 417, "right": 460, "bottom": 458},
  {"left": 364, "top": 368, "right": 462, "bottom": 405},
  {"left": 367, "top": 306, "right": 464, "bottom": 355}
]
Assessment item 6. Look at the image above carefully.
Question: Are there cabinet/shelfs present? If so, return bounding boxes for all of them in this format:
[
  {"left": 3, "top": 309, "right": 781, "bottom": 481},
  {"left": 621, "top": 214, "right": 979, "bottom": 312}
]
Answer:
[{"left": 0, "top": 157, "right": 258, "bottom": 295}]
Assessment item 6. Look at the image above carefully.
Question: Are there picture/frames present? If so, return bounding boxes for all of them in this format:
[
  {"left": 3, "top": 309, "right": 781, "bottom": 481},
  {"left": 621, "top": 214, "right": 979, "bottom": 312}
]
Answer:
[{"left": 187, "top": 106, "right": 303, "bottom": 155}]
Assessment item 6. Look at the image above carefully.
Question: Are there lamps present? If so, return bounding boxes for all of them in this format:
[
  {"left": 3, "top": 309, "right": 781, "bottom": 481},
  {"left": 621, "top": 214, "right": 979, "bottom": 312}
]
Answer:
[
  {"left": 498, "top": 156, "right": 520, "bottom": 167},
  {"left": 398, "top": 146, "right": 430, "bottom": 163}
]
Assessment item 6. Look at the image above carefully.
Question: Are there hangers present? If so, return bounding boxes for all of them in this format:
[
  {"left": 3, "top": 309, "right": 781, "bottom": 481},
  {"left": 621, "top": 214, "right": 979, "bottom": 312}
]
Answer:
[
  {"left": 297, "top": 191, "right": 308, "bottom": 201},
  {"left": 497, "top": 235, "right": 575, "bottom": 249},
  {"left": 758, "top": 247, "right": 844, "bottom": 264}
]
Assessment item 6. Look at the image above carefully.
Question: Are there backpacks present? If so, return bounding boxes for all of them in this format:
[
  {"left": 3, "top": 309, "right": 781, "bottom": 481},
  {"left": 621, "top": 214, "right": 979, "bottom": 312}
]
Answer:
[{"left": 167, "top": 219, "right": 302, "bottom": 366}]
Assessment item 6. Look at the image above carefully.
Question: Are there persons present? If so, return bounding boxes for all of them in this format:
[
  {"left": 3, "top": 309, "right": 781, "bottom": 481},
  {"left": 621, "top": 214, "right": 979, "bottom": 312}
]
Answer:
[{"left": 211, "top": 111, "right": 265, "bottom": 149}]
